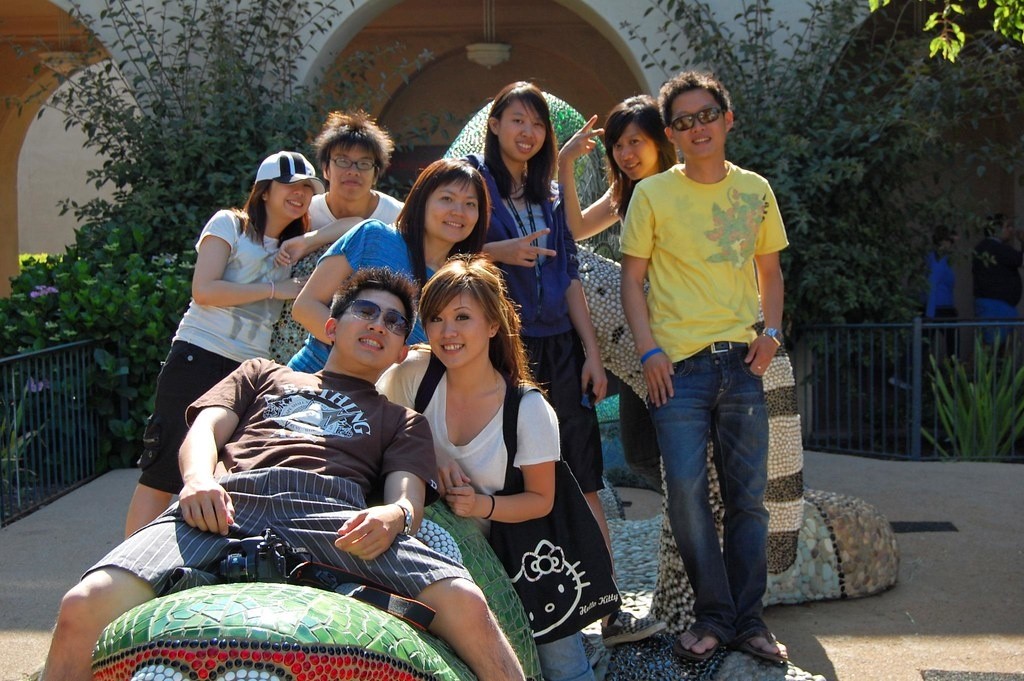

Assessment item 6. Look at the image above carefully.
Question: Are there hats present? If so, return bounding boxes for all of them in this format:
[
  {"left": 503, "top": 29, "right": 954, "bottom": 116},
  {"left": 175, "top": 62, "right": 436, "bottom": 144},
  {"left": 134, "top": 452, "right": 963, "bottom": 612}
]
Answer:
[{"left": 256, "top": 150, "right": 326, "bottom": 195}]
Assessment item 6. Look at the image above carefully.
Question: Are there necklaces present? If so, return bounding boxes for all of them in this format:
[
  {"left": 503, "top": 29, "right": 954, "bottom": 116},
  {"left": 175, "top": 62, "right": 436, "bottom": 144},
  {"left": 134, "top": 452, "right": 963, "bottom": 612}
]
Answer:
[
  {"left": 251, "top": 225, "right": 280, "bottom": 255},
  {"left": 504, "top": 188, "right": 545, "bottom": 311}
]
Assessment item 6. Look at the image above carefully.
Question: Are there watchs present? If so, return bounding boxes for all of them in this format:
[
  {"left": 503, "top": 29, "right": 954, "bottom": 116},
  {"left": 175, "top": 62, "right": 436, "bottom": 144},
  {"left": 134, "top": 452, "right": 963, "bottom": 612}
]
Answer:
[
  {"left": 388, "top": 502, "right": 413, "bottom": 536},
  {"left": 762, "top": 327, "right": 785, "bottom": 347}
]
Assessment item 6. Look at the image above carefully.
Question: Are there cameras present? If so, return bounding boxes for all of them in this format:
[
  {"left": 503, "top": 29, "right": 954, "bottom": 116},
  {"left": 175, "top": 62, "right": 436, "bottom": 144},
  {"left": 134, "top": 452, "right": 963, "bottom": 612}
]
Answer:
[{"left": 218, "top": 541, "right": 293, "bottom": 585}]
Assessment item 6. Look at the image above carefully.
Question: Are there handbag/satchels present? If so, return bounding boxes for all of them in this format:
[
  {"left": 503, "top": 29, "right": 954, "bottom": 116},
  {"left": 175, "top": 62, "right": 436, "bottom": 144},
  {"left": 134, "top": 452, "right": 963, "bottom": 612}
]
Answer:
[{"left": 489, "top": 380, "right": 622, "bottom": 647}]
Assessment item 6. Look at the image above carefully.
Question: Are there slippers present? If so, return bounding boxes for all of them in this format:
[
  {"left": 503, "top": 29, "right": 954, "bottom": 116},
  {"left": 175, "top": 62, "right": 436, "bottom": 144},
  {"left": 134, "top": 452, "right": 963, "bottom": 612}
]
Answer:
[
  {"left": 730, "top": 629, "right": 787, "bottom": 667},
  {"left": 674, "top": 627, "right": 720, "bottom": 662}
]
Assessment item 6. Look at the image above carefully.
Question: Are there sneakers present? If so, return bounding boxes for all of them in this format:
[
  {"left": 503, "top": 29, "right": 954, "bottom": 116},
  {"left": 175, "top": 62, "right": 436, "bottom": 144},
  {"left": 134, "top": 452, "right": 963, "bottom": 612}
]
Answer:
[
  {"left": 601, "top": 612, "right": 666, "bottom": 647},
  {"left": 581, "top": 633, "right": 600, "bottom": 666}
]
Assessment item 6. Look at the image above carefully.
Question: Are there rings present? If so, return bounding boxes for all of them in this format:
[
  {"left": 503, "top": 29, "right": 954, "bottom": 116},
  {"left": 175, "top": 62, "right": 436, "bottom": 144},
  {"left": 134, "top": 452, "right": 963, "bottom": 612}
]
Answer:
[{"left": 757, "top": 365, "right": 762, "bottom": 370}]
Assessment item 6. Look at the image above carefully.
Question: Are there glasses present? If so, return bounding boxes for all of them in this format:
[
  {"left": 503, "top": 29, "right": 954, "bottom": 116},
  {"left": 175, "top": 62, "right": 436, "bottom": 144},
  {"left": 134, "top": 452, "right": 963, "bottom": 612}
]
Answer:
[
  {"left": 328, "top": 157, "right": 377, "bottom": 170},
  {"left": 671, "top": 106, "right": 725, "bottom": 132},
  {"left": 339, "top": 299, "right": 411, "bottom": 336}
]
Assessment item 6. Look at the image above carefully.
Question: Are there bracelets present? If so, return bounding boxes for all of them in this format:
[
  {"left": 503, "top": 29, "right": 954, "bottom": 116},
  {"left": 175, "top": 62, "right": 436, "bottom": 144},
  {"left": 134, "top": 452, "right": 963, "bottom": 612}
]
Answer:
[
  {"left": 481, "top": 494, "right": 495, "bottom": 519},
  {"left": 640, "top": 347, "right": 663, "bottom": 365},
  {"left": 268, "top": 279, "right": 275, "bottom": 299}
]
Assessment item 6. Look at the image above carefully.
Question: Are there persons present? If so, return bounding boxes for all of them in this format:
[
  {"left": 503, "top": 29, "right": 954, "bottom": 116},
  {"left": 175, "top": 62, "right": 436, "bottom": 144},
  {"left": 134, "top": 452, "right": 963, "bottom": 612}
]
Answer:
[
  {"left": 971, "top": 214, "right": 1024, "bottom": 376},
  {"left": 125, "top": 81, "right": 616, "bottom": 580},
  {"left": 620, "top": 70, "right": 789, "bottom": 665},
  {"left": 924, "top": 226, "right": 959, "bottom": 363},
  {"left": 20, "top": 267, "right": 530, "bottom": 681},
  {"left": 555, "top": 95, "right": 682, "bottom": 242},
  {"left": 375, "top": 258, "right": 624, "bottom": 681}
]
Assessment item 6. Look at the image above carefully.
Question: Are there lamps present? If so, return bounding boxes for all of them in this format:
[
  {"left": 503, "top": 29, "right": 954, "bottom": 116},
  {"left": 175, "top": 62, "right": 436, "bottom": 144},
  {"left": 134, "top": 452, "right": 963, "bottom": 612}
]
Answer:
[{"left": 466, "top": 0, "right": 512, "bottom": 69}]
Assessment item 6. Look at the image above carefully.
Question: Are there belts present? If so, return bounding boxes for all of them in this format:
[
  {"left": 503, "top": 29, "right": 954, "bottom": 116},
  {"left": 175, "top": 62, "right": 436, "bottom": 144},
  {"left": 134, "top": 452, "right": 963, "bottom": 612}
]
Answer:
[{"left": 692, "top": 341, "right": 748, "bottom": 356}]
